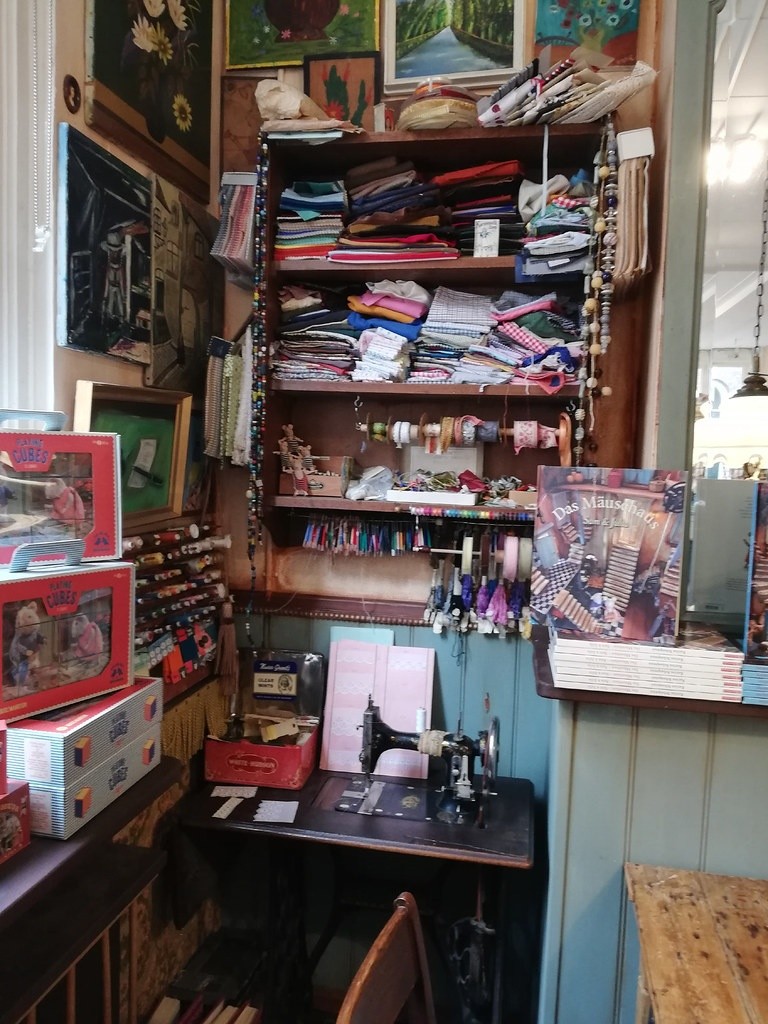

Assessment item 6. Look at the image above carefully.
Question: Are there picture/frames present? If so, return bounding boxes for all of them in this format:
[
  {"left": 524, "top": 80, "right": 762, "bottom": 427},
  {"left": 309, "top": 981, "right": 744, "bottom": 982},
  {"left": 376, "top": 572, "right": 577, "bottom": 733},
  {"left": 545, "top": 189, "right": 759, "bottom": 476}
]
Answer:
[
  {"left": 224, "top": 0, "right": 379, "bottom": 69},
  {"left": 72, "top": 380, "right": 194, "bottom": 538},
  {"left": 383, "top": 0, "right": 527, "bottom": 96},
  {"left": 303, "top": 52, "right": 380, "bottom": 132}
]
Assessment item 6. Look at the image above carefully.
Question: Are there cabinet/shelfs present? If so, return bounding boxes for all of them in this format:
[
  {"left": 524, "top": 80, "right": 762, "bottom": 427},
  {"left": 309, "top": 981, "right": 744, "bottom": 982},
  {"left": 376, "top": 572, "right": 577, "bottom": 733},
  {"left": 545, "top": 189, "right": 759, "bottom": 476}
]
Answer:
[{"left": 255, "top": 121, "right": 612, "bottom": 547}]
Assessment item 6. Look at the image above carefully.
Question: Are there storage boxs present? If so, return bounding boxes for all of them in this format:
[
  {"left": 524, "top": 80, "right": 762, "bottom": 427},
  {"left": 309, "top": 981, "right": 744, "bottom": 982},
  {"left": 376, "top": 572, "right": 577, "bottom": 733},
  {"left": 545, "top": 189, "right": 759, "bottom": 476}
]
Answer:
[
  {"left": 0, "top": 408, "right": 164, "bottom": 865},
  {"left": 281, "top": 456, "right": 354, "bottom": 497},
  {"left": 205, "top": 711, "right": 320, "bottom": 789}
]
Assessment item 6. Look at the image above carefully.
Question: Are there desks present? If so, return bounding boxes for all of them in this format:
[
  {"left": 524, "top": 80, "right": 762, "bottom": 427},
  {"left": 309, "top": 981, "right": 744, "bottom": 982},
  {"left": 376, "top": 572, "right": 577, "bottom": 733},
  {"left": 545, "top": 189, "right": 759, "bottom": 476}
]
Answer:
[
  {"left": 0, "top": 755, "right": 186, "bottom": 1024},
  {"left": 623, "top": 862, "right": 768, "bottom": 1024}
]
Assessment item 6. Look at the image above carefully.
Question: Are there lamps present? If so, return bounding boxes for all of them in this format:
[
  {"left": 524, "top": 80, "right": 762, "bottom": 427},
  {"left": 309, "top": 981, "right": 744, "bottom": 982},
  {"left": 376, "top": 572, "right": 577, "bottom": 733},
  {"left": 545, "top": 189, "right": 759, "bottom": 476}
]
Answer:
[{"left": 718, "top": 170, "right": 768, "bottom": 435}]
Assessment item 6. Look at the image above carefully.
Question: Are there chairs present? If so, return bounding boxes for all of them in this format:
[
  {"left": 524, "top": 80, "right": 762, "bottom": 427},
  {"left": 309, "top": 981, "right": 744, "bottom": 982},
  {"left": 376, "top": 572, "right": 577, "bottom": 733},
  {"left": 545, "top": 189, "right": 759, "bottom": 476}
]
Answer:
[{"left": 336, "top": 892, "right": 437, "bottom": 1024}]
